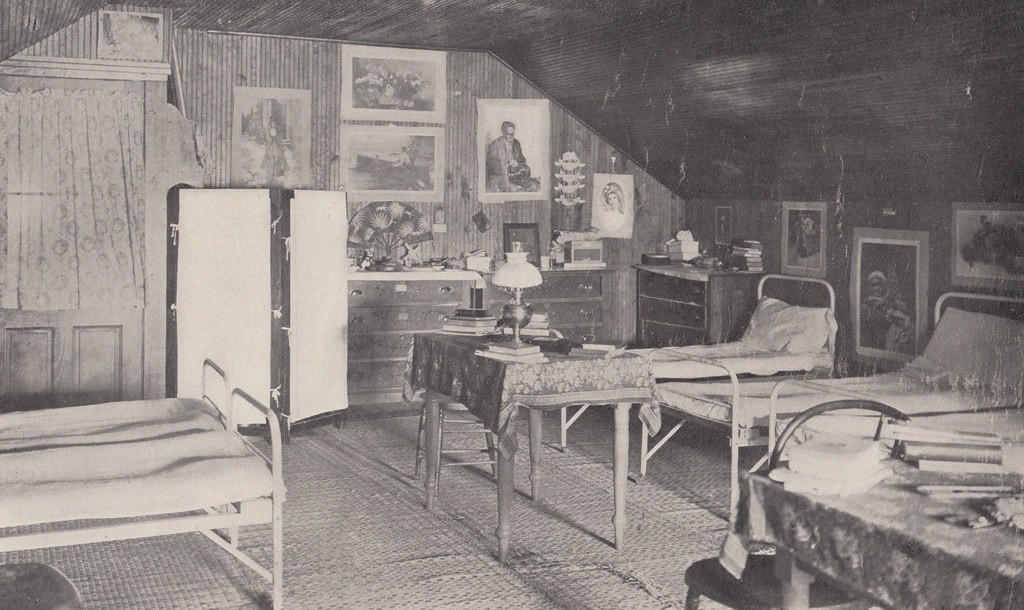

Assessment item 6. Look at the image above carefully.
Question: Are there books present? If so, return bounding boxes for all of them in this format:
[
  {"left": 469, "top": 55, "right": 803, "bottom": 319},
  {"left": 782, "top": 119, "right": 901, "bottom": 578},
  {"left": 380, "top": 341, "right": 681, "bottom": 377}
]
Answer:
[
  {"left": 784, "top": 419, "right": 1014, "bottom": 498},
  {"left": 665, "top": 230, "right": 699, "bottom": 262},
  {"left": 730, "top": 239, "right": 765, "bottom": 273}
]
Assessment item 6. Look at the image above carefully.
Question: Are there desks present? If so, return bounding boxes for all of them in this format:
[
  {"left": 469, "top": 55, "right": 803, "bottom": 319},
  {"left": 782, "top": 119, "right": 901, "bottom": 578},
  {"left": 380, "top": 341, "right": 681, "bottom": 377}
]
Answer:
[
  {"left": 718, "top": 460, "right": 1024, "bottom": 610},
  {"left": 402, "top": 333, "right": 665, "bottom": 565}
]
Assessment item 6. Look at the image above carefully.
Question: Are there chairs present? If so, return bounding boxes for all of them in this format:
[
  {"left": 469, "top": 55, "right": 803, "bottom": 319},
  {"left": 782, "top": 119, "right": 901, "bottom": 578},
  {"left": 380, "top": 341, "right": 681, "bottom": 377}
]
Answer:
[{"left": 683, "top": 398, "right": 916, "bottom": 609}]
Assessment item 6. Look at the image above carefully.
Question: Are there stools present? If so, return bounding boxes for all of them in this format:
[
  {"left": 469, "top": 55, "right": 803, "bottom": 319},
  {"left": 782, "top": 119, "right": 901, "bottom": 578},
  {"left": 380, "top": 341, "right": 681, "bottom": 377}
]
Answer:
[{"left": 411, "top": 391, "right": 497, "bottom": 496}]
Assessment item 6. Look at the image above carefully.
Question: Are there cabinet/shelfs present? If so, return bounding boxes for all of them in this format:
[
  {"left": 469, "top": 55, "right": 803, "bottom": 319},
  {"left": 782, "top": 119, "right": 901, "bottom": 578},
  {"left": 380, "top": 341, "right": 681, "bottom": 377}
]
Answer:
[
  {"left": 348, "top": 268, "right": 486, "bottom": 404},
  {"left": 469, "top": 270, "right": 619, "bottom": 343},
  {"left": 631, "top": 263, "right": 767, "bottom": 349}
]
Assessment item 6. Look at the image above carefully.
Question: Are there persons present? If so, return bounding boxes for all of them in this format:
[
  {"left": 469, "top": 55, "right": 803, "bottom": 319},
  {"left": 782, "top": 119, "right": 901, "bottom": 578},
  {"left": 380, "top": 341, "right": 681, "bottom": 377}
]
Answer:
[
  {"left": 599, "top": 186, "right": 626, "bottom": 230},
  {"left": 486, "top": 120, "right": 540, "bottom": 191}
]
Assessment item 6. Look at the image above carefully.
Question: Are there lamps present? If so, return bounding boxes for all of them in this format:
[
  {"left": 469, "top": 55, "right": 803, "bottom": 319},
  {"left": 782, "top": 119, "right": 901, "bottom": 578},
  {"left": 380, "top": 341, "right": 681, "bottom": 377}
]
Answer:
[{"left": 491, "top": 251, "right": 543, "bottom": 344}]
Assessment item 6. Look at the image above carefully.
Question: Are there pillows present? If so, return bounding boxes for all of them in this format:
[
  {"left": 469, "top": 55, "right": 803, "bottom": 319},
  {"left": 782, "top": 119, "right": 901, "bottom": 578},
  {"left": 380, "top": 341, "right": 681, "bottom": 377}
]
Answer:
[{"left": 740, "top": 295, "right": 837, "bottom": 357}]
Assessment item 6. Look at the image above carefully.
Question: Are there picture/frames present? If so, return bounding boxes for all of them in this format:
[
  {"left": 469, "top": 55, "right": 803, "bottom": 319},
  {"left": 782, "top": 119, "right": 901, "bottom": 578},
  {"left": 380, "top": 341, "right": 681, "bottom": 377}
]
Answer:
[{"left": 503, "top": 223, "right": 541, "bottom": 268}]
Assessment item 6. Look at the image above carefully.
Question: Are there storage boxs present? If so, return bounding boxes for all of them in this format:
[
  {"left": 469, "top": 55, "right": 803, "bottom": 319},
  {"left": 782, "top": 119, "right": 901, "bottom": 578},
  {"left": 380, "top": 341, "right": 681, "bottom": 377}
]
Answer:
[
  {"left": 466, "top": 255, "right": 491, "bottom": 270},
  {"left": 563, "top": 240, "right": 603, "bottom": 262}
]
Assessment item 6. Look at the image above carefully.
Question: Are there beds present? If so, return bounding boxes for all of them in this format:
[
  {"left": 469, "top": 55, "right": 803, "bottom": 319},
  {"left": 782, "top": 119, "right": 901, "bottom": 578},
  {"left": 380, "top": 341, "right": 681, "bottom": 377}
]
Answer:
[
  {"left": 0, "top": 357, "right": 286, "bottom": 610},
  {"left": 549, "top": 274, "right": 838, "bottom": 455},
  {"left": 635, "top": 292, "right": 1024, "bottom": 530}
]
